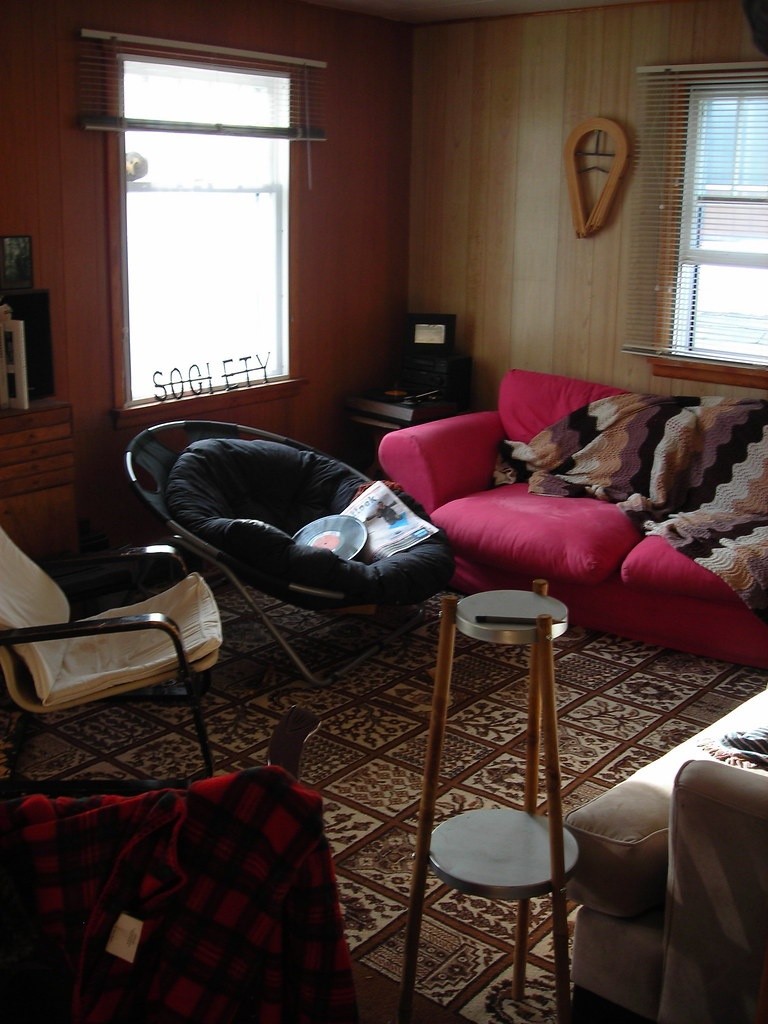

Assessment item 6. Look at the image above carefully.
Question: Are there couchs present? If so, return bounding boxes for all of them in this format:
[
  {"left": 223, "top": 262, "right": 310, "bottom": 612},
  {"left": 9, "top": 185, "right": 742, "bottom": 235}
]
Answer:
[
  {"left": 379, "top": 368, "right": 768, "bottom": 673},
  {"left": 564, "top": 685, "right": 768, "bottom": 1024}
]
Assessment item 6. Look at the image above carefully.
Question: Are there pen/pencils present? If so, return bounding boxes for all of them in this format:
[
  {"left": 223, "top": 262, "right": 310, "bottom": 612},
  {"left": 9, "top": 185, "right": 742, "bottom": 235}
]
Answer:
[{"left": 475, "top": 615, "right": 566, "bottom": 626}]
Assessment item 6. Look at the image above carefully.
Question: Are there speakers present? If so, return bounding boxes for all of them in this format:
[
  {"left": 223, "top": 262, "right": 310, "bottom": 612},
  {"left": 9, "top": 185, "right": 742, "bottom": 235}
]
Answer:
[{"left": 0, "top": 287, "right": 56, "bottom": 401}]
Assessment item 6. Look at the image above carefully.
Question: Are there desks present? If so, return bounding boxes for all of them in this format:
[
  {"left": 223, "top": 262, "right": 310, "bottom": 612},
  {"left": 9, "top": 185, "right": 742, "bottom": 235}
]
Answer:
[{"left": 344, "top": 407, "right": 471, "bottom": 480}]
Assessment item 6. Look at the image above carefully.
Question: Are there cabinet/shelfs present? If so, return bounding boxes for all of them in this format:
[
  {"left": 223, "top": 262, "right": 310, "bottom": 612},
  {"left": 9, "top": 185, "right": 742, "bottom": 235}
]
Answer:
[{"left": 399, "top": 575, "right": 573, "bottom": 1024}]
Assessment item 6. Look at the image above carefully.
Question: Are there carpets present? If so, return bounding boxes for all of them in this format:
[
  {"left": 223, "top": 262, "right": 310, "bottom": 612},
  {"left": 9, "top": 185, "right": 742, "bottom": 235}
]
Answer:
[{"left": 0, "top": 567, "right": 768, "bottom": 1024}]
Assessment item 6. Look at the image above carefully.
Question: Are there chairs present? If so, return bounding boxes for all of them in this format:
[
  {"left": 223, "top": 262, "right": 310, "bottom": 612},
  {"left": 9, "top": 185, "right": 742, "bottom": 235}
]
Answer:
[
  {"left": 0, "top": 526, "right": 223, "bottom": 800},
  {"left": 124, "top": 420, "right": 456, "bottom": 686}
]
